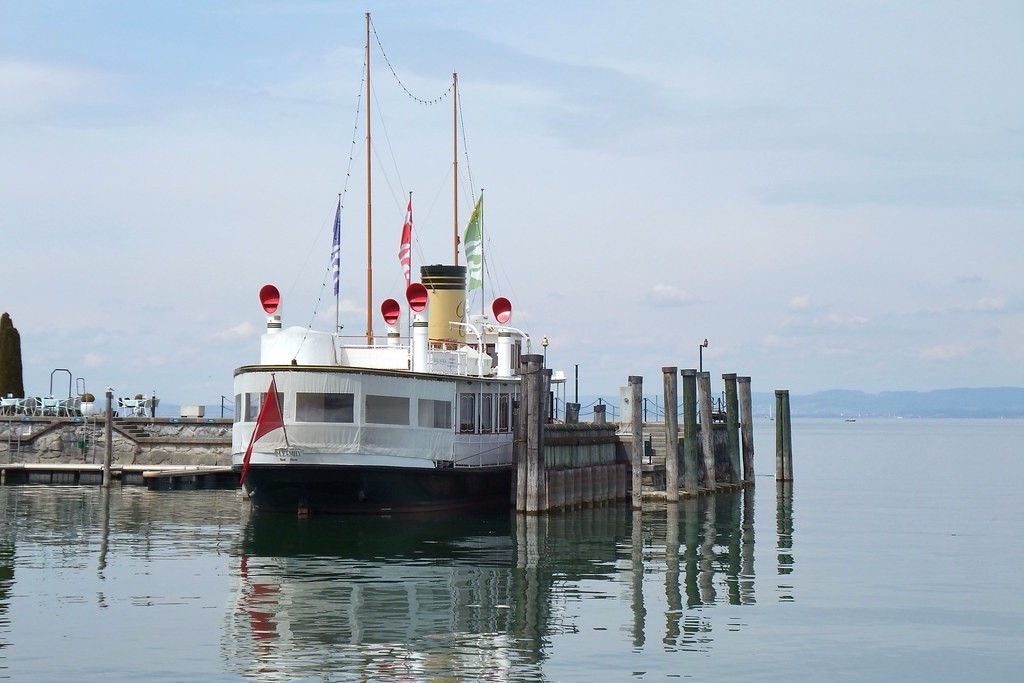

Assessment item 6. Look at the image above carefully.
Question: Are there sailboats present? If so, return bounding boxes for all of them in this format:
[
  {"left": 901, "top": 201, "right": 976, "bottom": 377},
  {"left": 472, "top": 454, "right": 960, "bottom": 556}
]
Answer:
[{"left": 769, "top": 403, "right": 774, "bottom": 420}]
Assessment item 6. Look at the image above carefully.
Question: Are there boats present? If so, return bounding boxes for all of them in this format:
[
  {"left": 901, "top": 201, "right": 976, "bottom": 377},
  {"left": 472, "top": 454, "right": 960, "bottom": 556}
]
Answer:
[
  {"left": 229, "top": 11, "right": 567, "bottom": 511},
  {"left": 846, "top": 419, "right": 856, "bottom": 422}
]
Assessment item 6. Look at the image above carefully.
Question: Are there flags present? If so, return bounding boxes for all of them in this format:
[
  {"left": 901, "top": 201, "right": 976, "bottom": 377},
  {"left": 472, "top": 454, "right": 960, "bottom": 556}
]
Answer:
[
  {"left": 398, "top": 200, "right": 412, "bottom": 288},
  {"left": 240, "top": 382, "right": 284, "bottom": 484},
  {"left": 463, "top": 194, "right": 485, "bottom": 291},
  {"left": 330, "top": 202, "right": 340, "bottom": 295}
]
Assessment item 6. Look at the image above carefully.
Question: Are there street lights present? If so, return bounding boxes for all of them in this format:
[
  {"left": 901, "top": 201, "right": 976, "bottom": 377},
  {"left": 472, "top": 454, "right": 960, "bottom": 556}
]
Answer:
[{"left": 699, "top": 338, "right": 708, "bottom": 370}]
